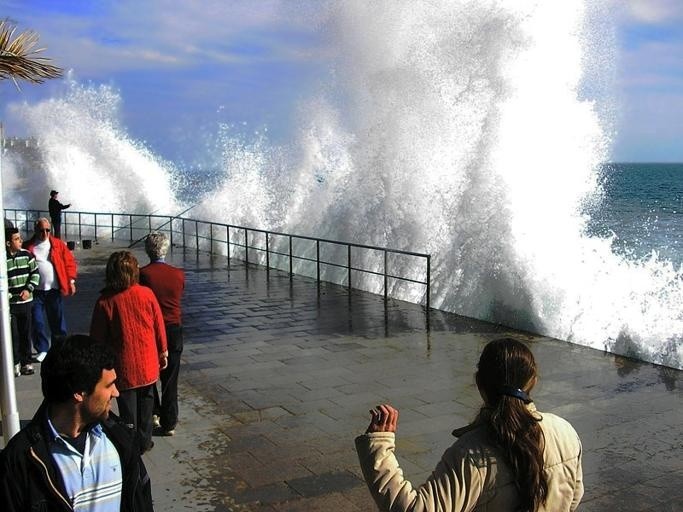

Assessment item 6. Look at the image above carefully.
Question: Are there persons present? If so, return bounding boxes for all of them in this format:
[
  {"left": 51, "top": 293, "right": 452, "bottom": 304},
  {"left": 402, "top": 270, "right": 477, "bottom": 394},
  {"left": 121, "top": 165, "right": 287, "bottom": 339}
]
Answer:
[
  {"left": 88, "top": 250, "right": 169, "bottom": 456},
  {"left": 355, "top": 339, "right": 585, "bottom": 512},
  {"left": 1, "top": 334, "right": 155, "bottom": 512},
  {"left": 140, "top": 229, "right": 186, "bottom": 438},
  {"left": 4, "top": 189, "right": 78, "bottom": 374}
]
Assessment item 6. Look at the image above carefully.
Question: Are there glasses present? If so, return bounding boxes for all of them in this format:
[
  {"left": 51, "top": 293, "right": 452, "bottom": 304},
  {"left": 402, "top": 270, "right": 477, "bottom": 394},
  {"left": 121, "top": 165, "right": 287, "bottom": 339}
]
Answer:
[{"left": 40, "top": 229, "right": 50, "bottom": 233}]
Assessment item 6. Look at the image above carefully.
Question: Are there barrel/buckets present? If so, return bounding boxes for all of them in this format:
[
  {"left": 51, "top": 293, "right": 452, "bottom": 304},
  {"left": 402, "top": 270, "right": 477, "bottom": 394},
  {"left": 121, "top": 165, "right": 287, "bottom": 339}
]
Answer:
[
  {"left": 81, "top": 239, "right": 92, "bottom": 249},
  {"left": 66, "top": 241, "right": 75, "bottom": 250}
]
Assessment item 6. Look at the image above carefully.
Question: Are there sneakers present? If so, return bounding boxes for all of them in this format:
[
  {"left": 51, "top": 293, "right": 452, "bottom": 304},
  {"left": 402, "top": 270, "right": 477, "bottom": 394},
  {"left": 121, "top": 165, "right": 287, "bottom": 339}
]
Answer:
[
  {"left": 153, "top": 415, "right": 161, "bottom": 429},
  {"left": 14, "top": 363, "right": 34, "bottom": 376},
  {"left": 36, "top": 352, "right": 47, "bottom": 362}
]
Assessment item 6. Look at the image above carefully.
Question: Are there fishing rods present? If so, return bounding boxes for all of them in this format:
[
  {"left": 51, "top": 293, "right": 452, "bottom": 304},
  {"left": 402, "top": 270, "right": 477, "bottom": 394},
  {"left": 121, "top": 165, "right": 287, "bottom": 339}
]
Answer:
[
  {"left": 93, "top": 210, "right": 159, "bottom": 243},
  {"left": 128, "top": 205, "right": 195, "bottom": 248}
]
